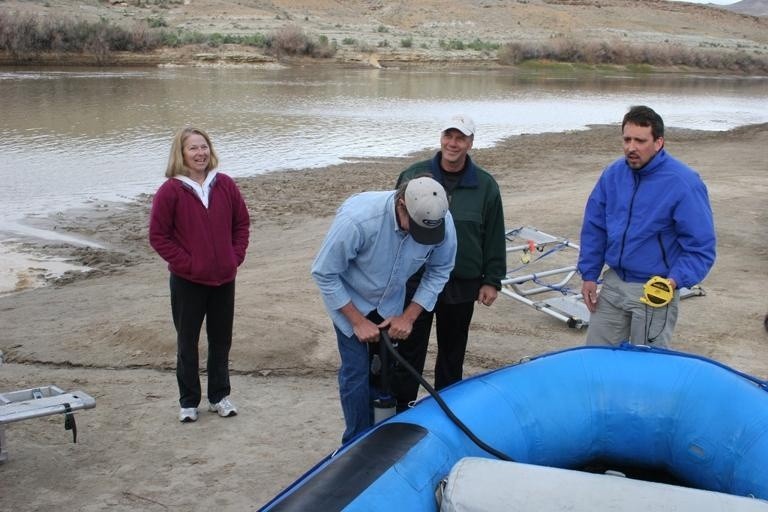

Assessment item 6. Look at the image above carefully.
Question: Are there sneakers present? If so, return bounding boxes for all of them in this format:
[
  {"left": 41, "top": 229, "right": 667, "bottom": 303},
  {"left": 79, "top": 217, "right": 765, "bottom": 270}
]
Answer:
[
  {"left": 208, "top": 395, "right": 238, "bottom": 417},
  {"left": 179, "top": 406, "right": 199, "bottom": 422}
]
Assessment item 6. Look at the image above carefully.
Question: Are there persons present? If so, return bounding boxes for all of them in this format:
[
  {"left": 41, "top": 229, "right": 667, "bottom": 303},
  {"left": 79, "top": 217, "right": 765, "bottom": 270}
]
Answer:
[
  {"left": 578, "top": 106, "right": 717, "bottom": 349},
  {"left": 393, "top": 114, "right": 506, "bottom": 418},
  {"left": 148, "top": 129, "right": 251, "bottom": 422},
  {"left": 313, "top": 173, "right": 459, "bottom": 445}
]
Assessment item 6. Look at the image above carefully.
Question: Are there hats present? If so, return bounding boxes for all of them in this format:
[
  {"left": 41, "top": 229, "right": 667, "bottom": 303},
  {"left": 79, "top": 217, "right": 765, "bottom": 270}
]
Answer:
[
  {"left": 403, "top": 175, "right": 448, "bottom": 246},
  {"left": 440, "top": 111, "right": 477, "bottom": 138}
]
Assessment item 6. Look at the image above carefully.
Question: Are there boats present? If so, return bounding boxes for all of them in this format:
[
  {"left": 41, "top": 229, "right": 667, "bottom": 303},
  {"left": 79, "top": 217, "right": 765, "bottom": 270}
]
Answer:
[{"left": 257, "top": 343, "right": 767, "bottom": 511}]
